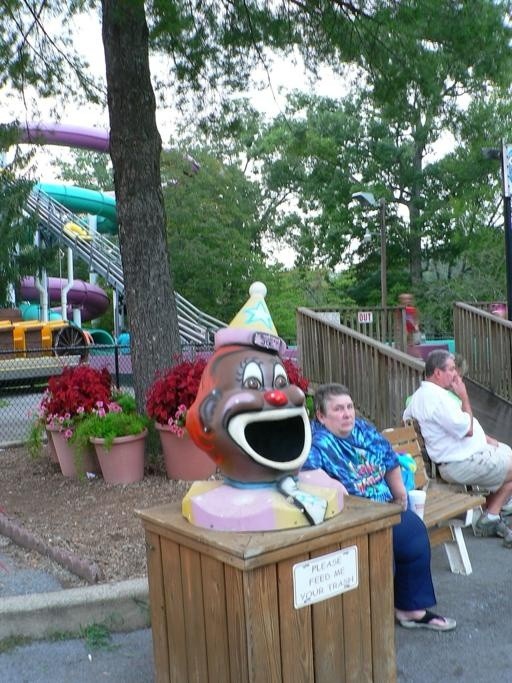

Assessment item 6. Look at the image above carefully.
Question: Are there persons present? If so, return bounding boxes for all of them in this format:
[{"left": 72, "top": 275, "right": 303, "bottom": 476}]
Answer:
[
  {"left": 399, "top": 350, "right": 512, "bottom": 538},
  {"left": 394, "top": 292, "right": 420, "bottom": 342},
  {"left": 302, "top": 381, "right": 457, "bottom": 632}
]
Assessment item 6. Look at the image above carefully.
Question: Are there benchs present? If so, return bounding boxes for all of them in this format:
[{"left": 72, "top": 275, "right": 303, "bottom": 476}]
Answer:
[
  {"left": 403, "top": 414, "right": 511, "bottom": 536},
  {"left": 375, "top": 421, "right": 488, "bottom": 576}
]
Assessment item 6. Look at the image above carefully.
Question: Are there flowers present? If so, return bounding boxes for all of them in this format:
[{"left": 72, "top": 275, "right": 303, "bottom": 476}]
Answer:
[
  {"left": 43, "top": 364, "right": 116, "bottom": 456},
  {"left": 65, "top": 389, "right": 149, "bottom": 479},
  {"left": 22, "top": 385, "right": 53, "bottom": 459},
  {"left": 141, "top": 350, "right": 208, "bottom": 437}
]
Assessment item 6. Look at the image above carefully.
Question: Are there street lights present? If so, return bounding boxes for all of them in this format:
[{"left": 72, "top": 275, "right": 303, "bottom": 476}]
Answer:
[
  {"left": 352, "top": 189, "right": 387, "bottom": 344},
  {"left": 480, "top": 145, "right": 511, "bottom": 372}
]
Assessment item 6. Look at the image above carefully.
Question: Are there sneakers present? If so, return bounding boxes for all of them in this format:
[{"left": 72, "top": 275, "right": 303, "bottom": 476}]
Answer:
[{"left": 475, "top": 502, "right": 512, "bottom": 548}]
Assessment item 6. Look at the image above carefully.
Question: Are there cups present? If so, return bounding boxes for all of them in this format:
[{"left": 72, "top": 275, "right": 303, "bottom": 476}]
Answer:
[{"left": 409, "top": 488, "right": 427, "bottom": 521}]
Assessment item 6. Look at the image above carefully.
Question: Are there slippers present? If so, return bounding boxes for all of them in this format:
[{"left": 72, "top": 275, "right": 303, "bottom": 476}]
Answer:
[{"left": 401, "top": 609, "right": 457, "bottom": 632}]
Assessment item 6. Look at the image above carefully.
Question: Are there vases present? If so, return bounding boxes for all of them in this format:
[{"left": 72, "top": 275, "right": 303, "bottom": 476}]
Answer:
[
  {"left": 87, "top": 428, "right": 151, "bottom": 484},
  {"left": 44, "top": 425, "right": 58, "bottom": 463},
  {"left": 45, "top": 419, "right": 103, "bottom": 476},
  {"left": 153, "top": 419, "right": 218, "bottom": 481}
]
